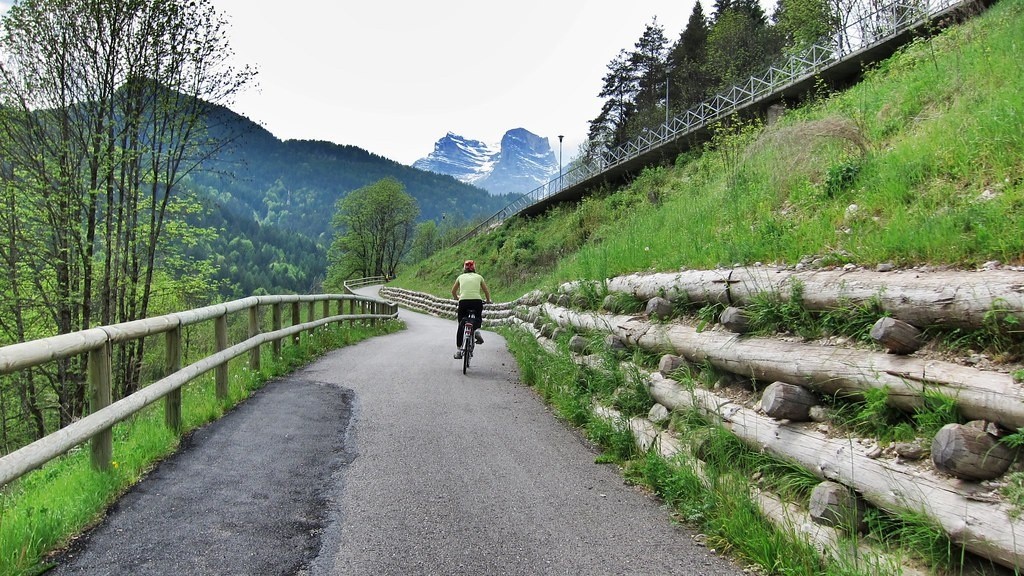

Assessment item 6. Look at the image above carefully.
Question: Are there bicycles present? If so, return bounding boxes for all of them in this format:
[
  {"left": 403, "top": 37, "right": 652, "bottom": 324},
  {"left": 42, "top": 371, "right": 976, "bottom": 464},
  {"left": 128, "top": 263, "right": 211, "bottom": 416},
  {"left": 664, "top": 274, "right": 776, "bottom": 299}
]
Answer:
[{"left": 452, "top": 297, "right": 493, "bottom": 374}]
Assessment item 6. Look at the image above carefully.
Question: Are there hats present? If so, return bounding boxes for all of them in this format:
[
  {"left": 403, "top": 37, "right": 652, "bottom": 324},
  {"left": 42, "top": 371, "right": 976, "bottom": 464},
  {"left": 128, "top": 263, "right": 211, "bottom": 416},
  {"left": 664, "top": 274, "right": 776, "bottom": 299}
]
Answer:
[{"left": 464, "top": 260, "right": 475, "bottom": 270}]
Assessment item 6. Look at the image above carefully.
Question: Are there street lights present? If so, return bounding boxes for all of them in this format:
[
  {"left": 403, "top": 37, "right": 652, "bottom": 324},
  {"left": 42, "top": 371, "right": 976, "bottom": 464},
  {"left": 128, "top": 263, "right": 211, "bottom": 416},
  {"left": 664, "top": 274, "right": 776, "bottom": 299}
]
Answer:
[
  {"left": 442, "top": 211, "right": 446, "bottom": 249},
  {"left": 557, "top": 134, "right": 565, "bottom": 191},
  {"left": 665, "top": 66, "right": 671, "bottom": 141}
]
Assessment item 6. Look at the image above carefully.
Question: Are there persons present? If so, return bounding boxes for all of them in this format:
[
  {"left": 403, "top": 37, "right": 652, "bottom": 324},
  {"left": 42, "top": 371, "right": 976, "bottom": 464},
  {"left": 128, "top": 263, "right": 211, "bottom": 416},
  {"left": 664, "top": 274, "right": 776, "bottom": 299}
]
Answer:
[
  {"left": 451, "top": 260, "right": 489, "bottom": 359},
  {"left": 386, "top": 273, "right": 389, "bottom": 283}
]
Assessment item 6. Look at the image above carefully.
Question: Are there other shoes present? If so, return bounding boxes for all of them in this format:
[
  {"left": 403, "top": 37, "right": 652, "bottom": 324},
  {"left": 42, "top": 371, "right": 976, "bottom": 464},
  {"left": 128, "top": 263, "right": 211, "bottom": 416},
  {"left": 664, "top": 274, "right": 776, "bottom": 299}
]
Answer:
[
  {"left": 475, "top": 332, "right": 484, "bottom": 343},
  {"left": 455, "top": 351, "right": 461, "bottom": 358}
]
